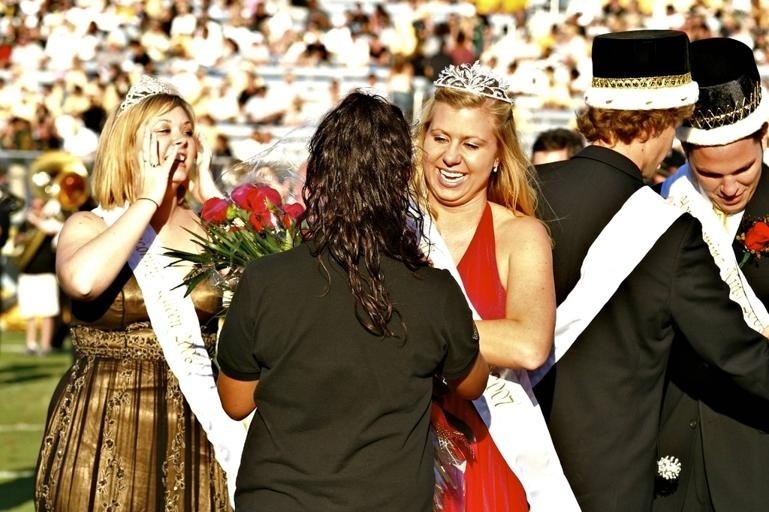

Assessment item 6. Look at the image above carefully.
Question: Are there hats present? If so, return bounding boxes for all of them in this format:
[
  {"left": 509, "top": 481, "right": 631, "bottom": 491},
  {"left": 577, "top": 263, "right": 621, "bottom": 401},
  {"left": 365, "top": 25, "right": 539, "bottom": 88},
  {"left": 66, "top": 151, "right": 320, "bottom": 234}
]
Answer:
[{"left": 584, "top": 30, "right": 765, "bottom": 147}]
